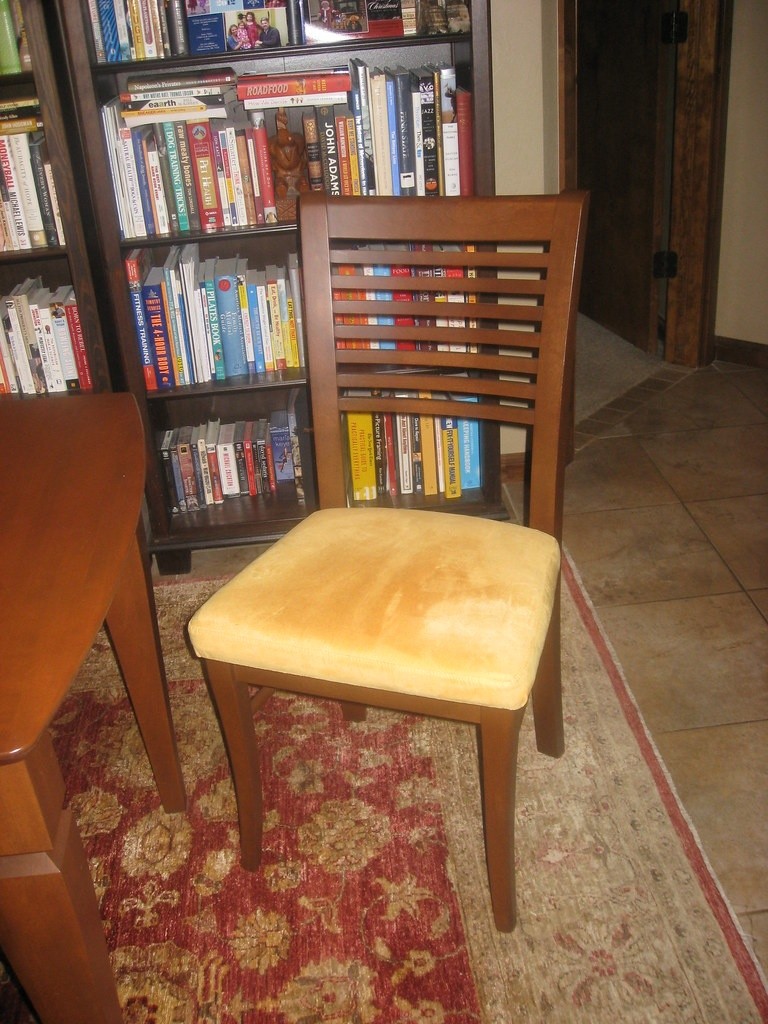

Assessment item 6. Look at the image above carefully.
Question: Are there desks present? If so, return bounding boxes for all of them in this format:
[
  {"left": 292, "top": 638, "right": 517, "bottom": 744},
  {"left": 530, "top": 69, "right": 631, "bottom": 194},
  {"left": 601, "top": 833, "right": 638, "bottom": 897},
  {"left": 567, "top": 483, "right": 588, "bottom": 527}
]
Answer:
[{"left": 1, "top": 389, "right": 189, "bottom": 1024}]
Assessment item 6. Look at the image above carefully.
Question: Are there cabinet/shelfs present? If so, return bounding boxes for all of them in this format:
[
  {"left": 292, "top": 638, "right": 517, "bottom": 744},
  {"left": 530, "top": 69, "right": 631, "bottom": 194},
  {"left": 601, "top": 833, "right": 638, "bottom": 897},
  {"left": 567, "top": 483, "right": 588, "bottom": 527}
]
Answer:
[
  {"left": 56, "top": 1, "right": 510, "bottom": 558},
  {"left": 0, "top": 1, "right": 112, "bottom": 394}
]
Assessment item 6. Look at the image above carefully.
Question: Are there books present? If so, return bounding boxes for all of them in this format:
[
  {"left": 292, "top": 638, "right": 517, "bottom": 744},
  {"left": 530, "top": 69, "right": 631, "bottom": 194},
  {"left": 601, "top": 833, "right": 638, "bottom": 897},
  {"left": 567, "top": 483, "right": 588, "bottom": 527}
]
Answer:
[
  {"left": 0, "top": 0, "right": 66, "bottom": 251},
  {"left": 99, "top": 56, "right": 476, "bottom": 242},
  {"left": 0, "top": 275, "right": 93, "bottom": 395},
  {"left": 158, "top": 388, "right": 483, "bottom": 521},
  {"left": 124, "top": 242, "right": 479, "bottom": 392},
  {"left": 84, "top": 0, "right": 312, "bottom": 64}
]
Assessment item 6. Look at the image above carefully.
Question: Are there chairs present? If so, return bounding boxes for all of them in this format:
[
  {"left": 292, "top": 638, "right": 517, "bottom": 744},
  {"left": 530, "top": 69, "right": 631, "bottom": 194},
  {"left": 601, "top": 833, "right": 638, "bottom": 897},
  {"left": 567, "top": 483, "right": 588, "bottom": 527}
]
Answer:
[{"left": 188, "top": 192, "right": 592, "bottom": 933}]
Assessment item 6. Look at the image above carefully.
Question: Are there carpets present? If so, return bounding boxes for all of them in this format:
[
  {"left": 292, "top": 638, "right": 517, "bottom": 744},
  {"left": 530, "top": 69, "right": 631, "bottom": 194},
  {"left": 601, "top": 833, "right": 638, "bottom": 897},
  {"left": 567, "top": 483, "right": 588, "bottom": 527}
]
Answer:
[{"left": 1, "top": 545, "right": 768, "bottom": 1024}]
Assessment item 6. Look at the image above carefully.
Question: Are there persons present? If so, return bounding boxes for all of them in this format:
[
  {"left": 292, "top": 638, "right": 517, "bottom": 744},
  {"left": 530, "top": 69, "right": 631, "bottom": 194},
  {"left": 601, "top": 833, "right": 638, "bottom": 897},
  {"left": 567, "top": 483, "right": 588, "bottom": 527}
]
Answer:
[
  {"left": 255, "top": 17, "right": 281, "bottom": 48},
  {"left": 237, "top": 21, "right": 251, "bottom": 50},
  {"left": 245, "top": 11, "right": 262, "bottom": 48},
  {"left": 318, "top": 0, "right": 362, "bottom": 33},
  {"left": 227, "top": 23, "right": 249, "bottom": 51}
]
